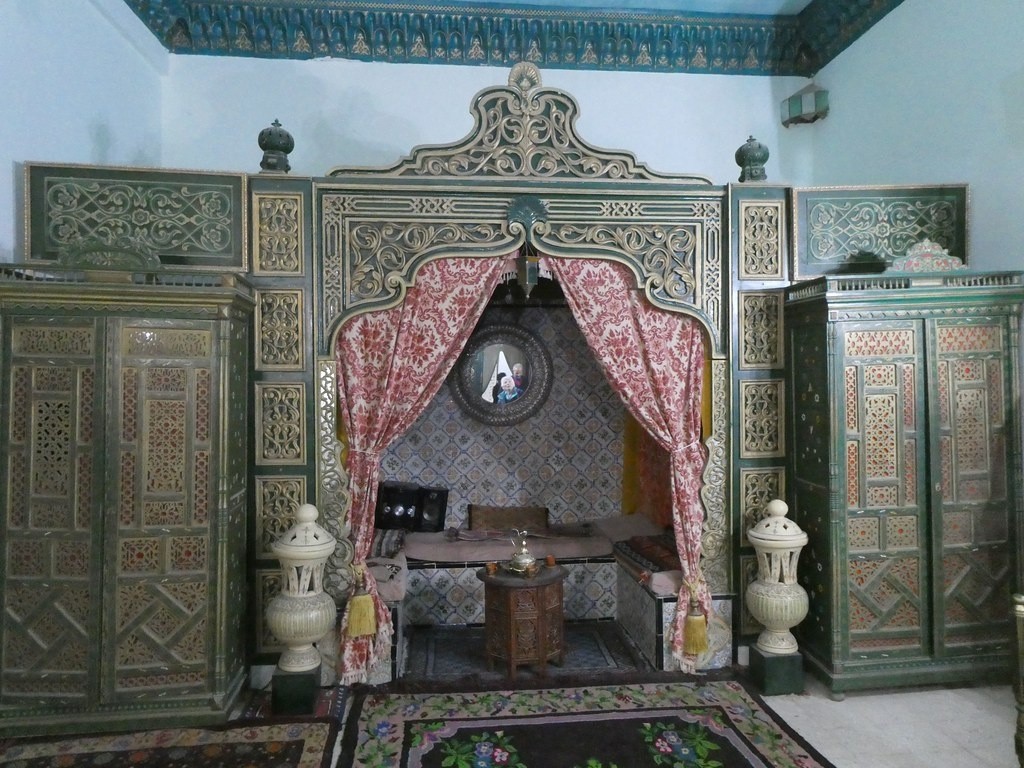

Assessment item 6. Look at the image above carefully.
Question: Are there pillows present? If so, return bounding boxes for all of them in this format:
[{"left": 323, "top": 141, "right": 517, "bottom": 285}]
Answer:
[{"left": 467, "top": 505, "right": 553, "bottom": 539}]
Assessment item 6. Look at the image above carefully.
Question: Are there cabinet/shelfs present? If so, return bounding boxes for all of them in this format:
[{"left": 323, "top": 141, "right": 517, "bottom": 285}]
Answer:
[
  {"left": 782, "top": 269, "right": 1023, "bottom": 702},
  {"left": 0, "top": 262, "right": 257, "bottom": 741}
]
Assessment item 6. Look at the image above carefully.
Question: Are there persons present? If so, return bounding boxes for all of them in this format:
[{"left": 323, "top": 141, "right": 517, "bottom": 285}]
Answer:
[
  {"left": 511, "top": 363, "right": 528, "bottom": 389},
  {"left": 498, "top": 376, "right": 523, "bottom": 404}
]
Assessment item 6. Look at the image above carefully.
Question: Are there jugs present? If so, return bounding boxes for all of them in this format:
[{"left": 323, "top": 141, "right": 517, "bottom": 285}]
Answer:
[{"left": 510, "top": 528, "right": 535, "bottom": 573}]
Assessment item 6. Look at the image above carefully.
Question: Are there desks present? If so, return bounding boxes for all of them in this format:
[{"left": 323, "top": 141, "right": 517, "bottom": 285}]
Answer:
[{"left": 475, "top": 564, "right": 570, "bottom": 679}]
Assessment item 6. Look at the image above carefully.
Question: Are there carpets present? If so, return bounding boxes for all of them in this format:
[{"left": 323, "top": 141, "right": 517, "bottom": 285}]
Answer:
[
  {"left": 403, "top": 627, "right": 647, "bottom": 681},
  {"left": 0, "top": 714, "right": 341, "bottom": 768},
  {"left": 335, "top": 668, "right": 837, "bottom": 768}
]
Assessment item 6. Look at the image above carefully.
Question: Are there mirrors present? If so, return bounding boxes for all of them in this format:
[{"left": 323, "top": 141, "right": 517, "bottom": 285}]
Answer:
[{"left": 447, "top": 322, "right": 553, "bottom": 427}]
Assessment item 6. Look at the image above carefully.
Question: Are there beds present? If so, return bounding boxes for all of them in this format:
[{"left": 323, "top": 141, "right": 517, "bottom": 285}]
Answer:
[{"left": 365, "top": 511, "right": 666, "bottom": 571}]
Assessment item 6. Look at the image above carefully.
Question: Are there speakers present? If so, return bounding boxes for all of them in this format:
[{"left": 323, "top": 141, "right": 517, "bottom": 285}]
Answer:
[{"left": 417, "top": 484, "right": 450, "bottom": 531}]
